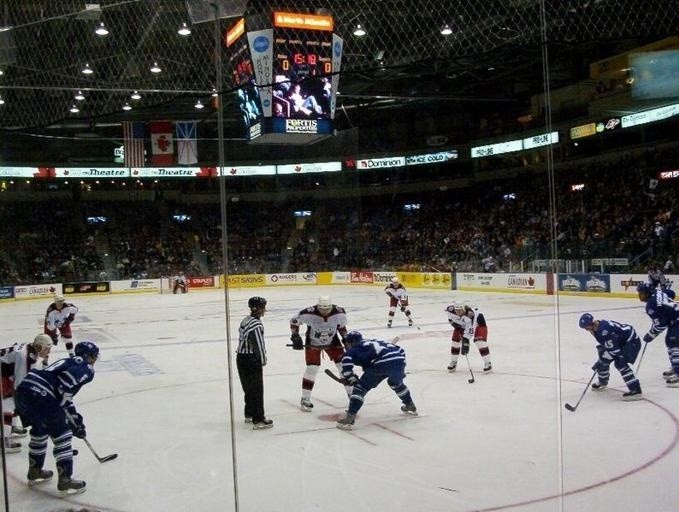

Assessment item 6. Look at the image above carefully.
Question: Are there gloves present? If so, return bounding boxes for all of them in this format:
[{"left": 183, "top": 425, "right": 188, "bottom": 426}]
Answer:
[
  {"left": 595, "top": 344, "right": 605, "bottom": 361},
  {"left": 70, "top": 423, "right": 87, "bottom": 440},
  {"left": 290, "top": 333, "right": 304, "bottom": 351},
  {"left": 346, "top": 374, "right": 361, "bottom": 385},
  {"left": 460, "top": 337, "right": 470, "bottom": 355},
  {"left": 591, "top": 360, "right": 604, "bottom": 372},
  {"left": 643, "top": 329, "right": 659, "bottom": 344},
  {"left": 67, "top": 409, "right": 84, "bottom": 426},
  {"left": 50, "top": 334, "right": 58, "bottom": 346},
  {"left": 448, "top": 318, "right": 462, "bottom": 331},
  {"left": 400, "top": 306, "right": 406, "bottom": 313}
]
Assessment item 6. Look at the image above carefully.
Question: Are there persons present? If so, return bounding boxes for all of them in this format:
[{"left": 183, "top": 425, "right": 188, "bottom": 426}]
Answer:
[
  {"left": 468, "top": 169, "right": 552, "bottom": 273},
  {"left": 1, "top": 198, "right": 107, "bottom": 285},
  {"left": 637, "top": 284, "right": 679, "bottom": 385},
  {"left": 1, "top": 334, "right": 52, "bottom": 450},
  {"left": 337, "top": 331, "right": 417, "bottom": 425},
  {"left": 384, "top": 277, "right": 413, "bottom": 328},
  {"left": 444, "top": 299, "right": 492, "bottom": 372},
  {"left": 14, "top": 341, "right": 99, "bottom": 491},
  {"left": 362, "top": 191, "right": 468, "bottom": 272},
  {"left": 579, "top": 313, "right": 642, "bottom": 399},
  {"left": 228, "top": 194, "right": 360, "bottom": 273},
  {"left": 553, "top": 142, "right": 679, "bottom": 290},
  {"left": 236, "top": 296, "right": 273, "bottom": 427},
  {"left": 109, "top": 199, "right": 223, "bottom": 278},
  {"left": 173, "top": 272, "right": 187, "bottom": 294},
  {"left": 275, "top": 62, "right": 332, "bottom": 120},
  {"left": 289, "top": 295, "right": 357, "bottom": 408},
  {"left": 43, "top": 296, "right": 79, "bottom": 369}
]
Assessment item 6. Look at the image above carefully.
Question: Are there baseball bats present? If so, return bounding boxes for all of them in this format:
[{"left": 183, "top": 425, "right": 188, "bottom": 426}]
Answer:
[{"left": 324, "top": 370, "right": 406, "bottom": 388}]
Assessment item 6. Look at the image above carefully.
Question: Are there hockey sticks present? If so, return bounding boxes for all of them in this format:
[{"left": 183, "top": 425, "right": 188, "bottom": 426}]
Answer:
[
  {"left": 460, "top": 333, "right": 475, "bottom": 383},
  {"left": 565, "top": 368, "right": 598, "bottom": 411},
  {"left": 286, "top": 336, "right": 399, "bottom": 350},
  {"left": 62, "top": 409, "right": 117, "bottom": 463},
  {"left": 398, "top": 302, "right": 420, "bottom": 329}
]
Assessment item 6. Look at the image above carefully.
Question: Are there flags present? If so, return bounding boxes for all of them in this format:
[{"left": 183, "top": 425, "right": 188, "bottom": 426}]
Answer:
[
  {"left": 122, "top": 120, "right": 145, "bottom": 167},
  {"left": 148, "top": 119, "right": 174, "bottom": 165},
  {"left": 176, "top": 120, "right": 198, "bottom": 166}
]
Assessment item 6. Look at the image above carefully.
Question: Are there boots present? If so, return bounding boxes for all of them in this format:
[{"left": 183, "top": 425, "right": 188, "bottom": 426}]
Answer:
[
  {"left": 482, "top": 361, "right": 494, "bottom": 372},
  {"left": 25, "top": 466, "right": 55, "bottom": 489},
  {"left": 661, "top": 364, "right": 678, "bottom": 379},
  {"left": 408, "top": 319, "right": 413, "bottom": 327},
  {"left": 336, "top": 412, "right": 357, "bottom": 431},
  {"left": 299, "top": 395, "right": 315, "bottom": 412},
  {"left": 253, "top": 416, "right": 274, "bottom": 430},
  {"left": 3, "top": 437, "right": 23, "bottom": 455},
  {"left": 387, "top": 320, "right": 392, "bottom": 328},
  {"left": 400, "top": 401, "right": 419, "bottom": 418},
  {"left": 620, "top": 385, "right": 643, "bottom": 402},
  {"left": 591, "top": 378, "right": 610, "bottom": 392},
  {"left": 54, "top": 474, "right": 87, "bottom": 498},
  {"left": 10, "top": 425, "right": 28, "bottom": 439},
  {"left": 41, "top": 359, "right": 49, "bottom": 369},
  {"left": 243, "top": 412, "right": 254, "bottom": 424},
  {"left": 665, "top": 374, "right": 679, "bottom": 388},
  {"left": 446, "top": 359, "right": 458, "bottom": 372}
]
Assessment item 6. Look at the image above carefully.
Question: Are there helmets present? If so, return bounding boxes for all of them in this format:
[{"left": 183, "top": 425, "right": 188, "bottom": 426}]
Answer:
[
  {"left": 341, "top": 330, "right": 363, "bottom": 349},
  {"left": 637, "top": 282, "right": 654, "bottom": 296},
  {"left": 247, "top": 295, "right": 267, "bottom": 316},
  {"left": 578, "top": 313, "right": 593, "bottom": 329},
  {"left": 53, "top": 293, "right": 66, "bottom": 307},
  {"left": 649, "top": 265, "right": 657, "bottom": 273},
  {"left": 318, "top": 296, "right": 333, "bottom": 312},
  {"left": 453, "top": 300, "right": 467, "bottom": 314},
  {"left": 392, "top": 277, "right": 400, "bottom": 287},
  {"left": 33, "top": 333, "right": 55, "bottom": 357},
  {"left": 74, "top": 341, "right": 100, "bottom": 364}
]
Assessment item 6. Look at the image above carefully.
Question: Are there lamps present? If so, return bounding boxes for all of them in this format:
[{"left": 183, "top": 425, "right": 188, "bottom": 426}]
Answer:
[
  {"left": 179, "top": 19, "right": 191, "bottom": 36},
  {"left": 75, "top": 91, "right": 86, "bottom": 100},
  {"left": 149, "top": 62, "right": 162, "bottom": 73},
  {"left": 81, "top": 64, "right": 94, "bottom": 74},
  {"left": 94, "top": 21, "right": 111, "bottom": 37},
  {"left": 440, "top": 23, "right": 454, "bottom": 36},
  {"left": 196, "top": 100, "right": 204, "bottom": 109},
  {"left": 354, "top": 25, "right": 366, "bottom": 36},
  {"left": 69, "top": 105, "right": 80, "bottom": 113},
  {"left": 131, "top": 91, "right": 142, "bottom": 99},
  {"left": 124, "top": 102, "right": 133, "bottom": 110}
]
